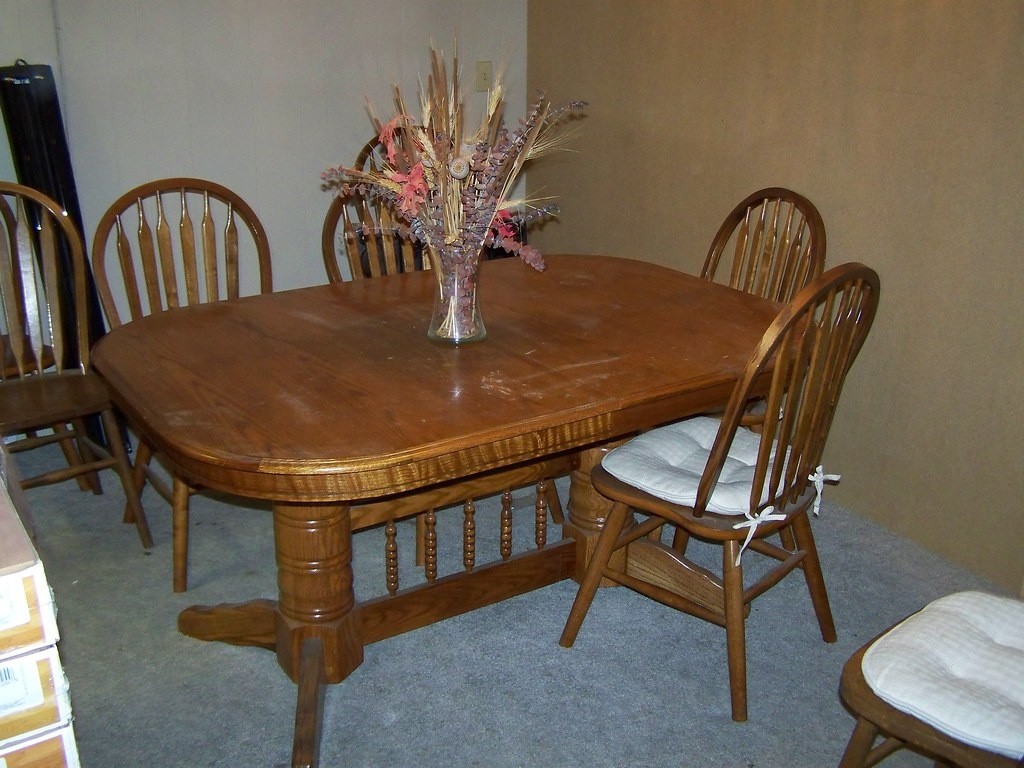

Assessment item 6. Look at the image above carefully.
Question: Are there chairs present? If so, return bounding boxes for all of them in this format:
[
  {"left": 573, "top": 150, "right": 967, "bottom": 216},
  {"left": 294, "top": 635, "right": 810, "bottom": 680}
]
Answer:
[
  {"left": 351, "top": 125, "right": 453, "bottom": 225},
  {"left": 95, "top": 178, "right": 273, "bottom": 594},
  {"left": 0, "top": 183, "right": 151, "bottom": 550},
  {"left": 557, "top": 261, "right": 881, "bottom": 722},
  {"left": 837, "top": 588, "right": 1024, "bottom": 768},
  {"left": 322, "top": 174, "right": 429, "bottom": 283},
  {"left": 699, "top": 185, "right": 828, "bottom": 433}
]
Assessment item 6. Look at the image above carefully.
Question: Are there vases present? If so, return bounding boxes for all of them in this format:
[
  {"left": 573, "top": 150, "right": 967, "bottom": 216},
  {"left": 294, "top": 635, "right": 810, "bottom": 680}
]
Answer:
[{"left": 428, "top": 244, "right": 487, "bottom": 340}]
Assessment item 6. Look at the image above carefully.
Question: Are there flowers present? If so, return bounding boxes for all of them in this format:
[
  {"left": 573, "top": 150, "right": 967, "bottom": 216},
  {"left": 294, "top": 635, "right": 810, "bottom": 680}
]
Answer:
[{"left": 316, "top": 30, "right": 590, "bottom": 271}]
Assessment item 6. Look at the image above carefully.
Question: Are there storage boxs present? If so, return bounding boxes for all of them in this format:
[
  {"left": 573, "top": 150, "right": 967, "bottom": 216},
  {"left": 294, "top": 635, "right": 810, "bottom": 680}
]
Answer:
[
  {"left": 1, "top": 643, "right": 73, "bottom": 741},
  {"left": 0, "top": 471, "right": 61, "bottom": 660},
  {"left": 0, "top": 726, "right": 79, "bottom": 768}
]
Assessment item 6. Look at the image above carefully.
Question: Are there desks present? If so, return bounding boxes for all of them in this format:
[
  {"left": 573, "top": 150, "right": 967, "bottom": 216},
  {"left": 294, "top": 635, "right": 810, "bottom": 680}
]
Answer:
[{"left": 87, "top": 254, "right": 815, "bottom": 768}]
